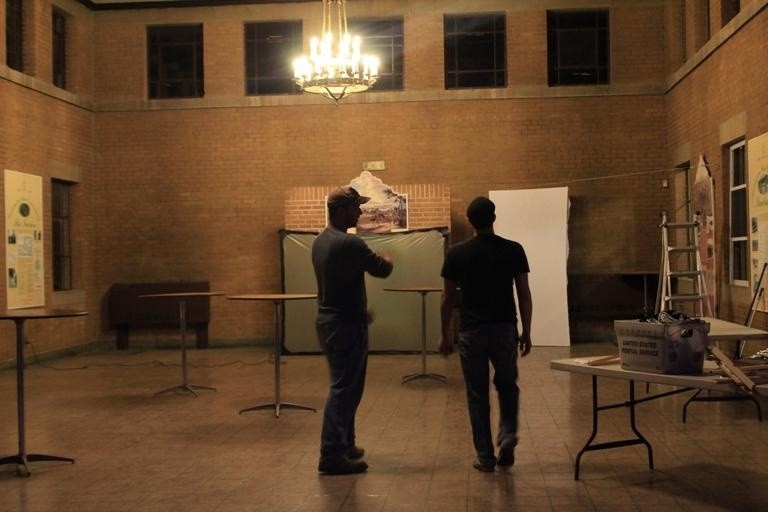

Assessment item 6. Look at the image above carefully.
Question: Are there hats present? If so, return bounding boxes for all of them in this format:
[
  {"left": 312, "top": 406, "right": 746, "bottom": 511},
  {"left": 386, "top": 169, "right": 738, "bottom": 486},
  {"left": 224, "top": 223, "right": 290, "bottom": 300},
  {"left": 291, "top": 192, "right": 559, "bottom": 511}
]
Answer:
[{"left": 326, "top": 185, "right": 371, "bottom": 208}]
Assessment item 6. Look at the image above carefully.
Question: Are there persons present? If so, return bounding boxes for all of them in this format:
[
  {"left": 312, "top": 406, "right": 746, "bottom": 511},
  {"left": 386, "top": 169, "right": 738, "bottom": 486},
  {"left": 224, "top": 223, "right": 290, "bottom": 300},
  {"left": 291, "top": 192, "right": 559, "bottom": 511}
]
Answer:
[
  {"left": 312, "top": 187, "right": 393, "bottom": 474},
  {"left": 437, "top": 196, "right": 532, "bottom": 472}
]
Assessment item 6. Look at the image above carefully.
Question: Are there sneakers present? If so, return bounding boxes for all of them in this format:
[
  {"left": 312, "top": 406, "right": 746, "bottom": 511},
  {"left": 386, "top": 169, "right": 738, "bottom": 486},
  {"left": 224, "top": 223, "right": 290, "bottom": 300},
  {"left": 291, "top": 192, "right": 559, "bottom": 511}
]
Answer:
[
  {"left": 498, "top": 441, "right": 514, "bottom": 467},
  {"left": 473, "top": 454, "right": 497, "bottom": 472}
]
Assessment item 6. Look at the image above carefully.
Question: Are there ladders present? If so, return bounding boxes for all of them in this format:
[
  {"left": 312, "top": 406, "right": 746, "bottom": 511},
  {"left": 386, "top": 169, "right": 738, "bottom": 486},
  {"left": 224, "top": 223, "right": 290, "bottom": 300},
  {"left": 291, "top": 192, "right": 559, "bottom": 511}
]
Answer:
[{"left": 653, "top": 210, "right": 713, "bottom": 320}]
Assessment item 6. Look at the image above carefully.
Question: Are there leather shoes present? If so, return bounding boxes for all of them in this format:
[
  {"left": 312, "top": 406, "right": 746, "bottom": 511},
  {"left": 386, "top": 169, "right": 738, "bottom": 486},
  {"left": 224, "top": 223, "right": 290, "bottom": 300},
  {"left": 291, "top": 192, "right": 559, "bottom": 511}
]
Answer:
[
  {"left": 345, "top": 445, "right": 365, "bottom": 458},
  {"left": 318, "top": 456, "right": 369, "bottom": 476}
]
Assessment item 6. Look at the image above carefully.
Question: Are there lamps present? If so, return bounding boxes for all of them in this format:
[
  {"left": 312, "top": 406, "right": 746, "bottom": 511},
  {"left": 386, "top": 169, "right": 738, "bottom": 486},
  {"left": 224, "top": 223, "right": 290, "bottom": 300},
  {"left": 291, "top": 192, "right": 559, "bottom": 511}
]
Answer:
[{"left": 291, "top": 0, "right": 382, "bottom": 107}]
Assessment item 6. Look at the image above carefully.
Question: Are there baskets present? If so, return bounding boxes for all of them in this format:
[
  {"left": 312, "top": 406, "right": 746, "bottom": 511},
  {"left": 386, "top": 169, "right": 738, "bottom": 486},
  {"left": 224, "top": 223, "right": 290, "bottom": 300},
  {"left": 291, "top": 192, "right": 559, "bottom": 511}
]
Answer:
[{"left": 611, "top": 313, "right": 712, "bottom": 377}]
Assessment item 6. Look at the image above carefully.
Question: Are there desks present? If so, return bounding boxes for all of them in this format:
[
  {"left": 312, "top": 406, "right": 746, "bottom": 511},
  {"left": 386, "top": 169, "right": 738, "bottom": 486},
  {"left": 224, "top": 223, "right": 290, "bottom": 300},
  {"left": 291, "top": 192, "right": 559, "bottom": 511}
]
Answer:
[
  {"left": 645, "top": 316, "right": 768, "bottom": 424},
  {"left": 138, "top": 292, "right": 226, "bottom": 398},
  {"left": 1, "top": 309, "right": 88, "bottom": 477},
  {"left": 567, "top": 272, "right": 660, "bottom": 342},
  {"left": 106, "top": 281, "right": 210, "bottom": 351},
  {"left": 549, "top": 356, "right": 767, "bottom": 480},
  {"left": 229, "top": 294, "right": 319, "bottom": 418},
  {"left": 382, "top": 287, "right": 450, "bottom": 384}
]
